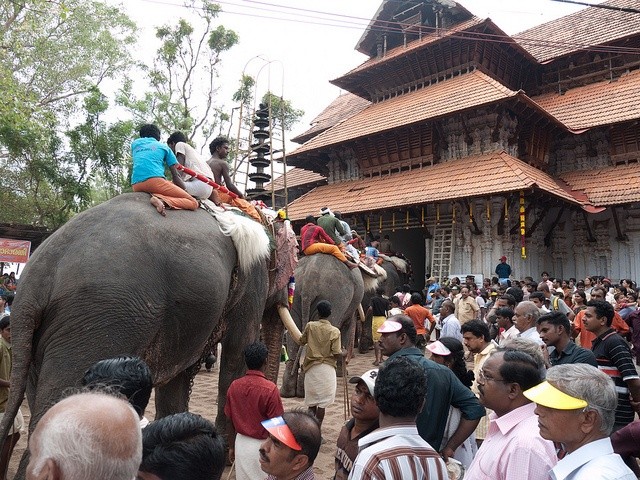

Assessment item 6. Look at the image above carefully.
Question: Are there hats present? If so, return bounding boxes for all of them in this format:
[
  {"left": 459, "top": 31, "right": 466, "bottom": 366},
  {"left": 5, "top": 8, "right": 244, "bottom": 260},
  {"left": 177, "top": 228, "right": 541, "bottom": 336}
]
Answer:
[
  {"left": 425, "top": 338, "right": 467, "bottom": 358},
  {"left": 259, "top": 414, "right": 321, "bottom": 457},
  {"left": 350, "top": 369, "right": 379, "bottom": 397},
  {"left": 499, "top": 256, "right": 507, "bottom": 261},
  {"left": 523, "top": 381, "right": 619, "bottom": 414},
  {"left": 377, "top": 319, "right": 414, "bottom": 335}
]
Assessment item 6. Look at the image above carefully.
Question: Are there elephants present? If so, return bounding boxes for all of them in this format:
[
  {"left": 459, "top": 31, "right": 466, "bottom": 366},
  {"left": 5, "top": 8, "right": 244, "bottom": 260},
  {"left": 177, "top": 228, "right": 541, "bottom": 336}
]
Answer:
[
  {"left": 0, "top": 192, "right": 304, "bottom": 480},
  {"left": 355, "top": 258, "right": 407, "bottom": 352},
  {"left": 279, "top": 252, "right": 366, "bottom": 396}
]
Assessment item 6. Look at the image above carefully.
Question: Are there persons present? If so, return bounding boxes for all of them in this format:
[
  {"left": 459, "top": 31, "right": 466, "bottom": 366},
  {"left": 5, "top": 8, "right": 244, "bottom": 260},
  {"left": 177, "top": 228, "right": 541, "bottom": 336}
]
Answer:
[
  {"left": 300, "top": 215, "right": 360, "bottom": 270},
  {"left": 81, "top": 355, "right": 151, "bottom": 436},
  {"left": 24, "top": 389, "right": 144, "bottom": 479},
  {"left": 495, "top": 256, "right": 511, "bottom": 283},
  {"left": 536, "top": 311, "right": 597, "bottom": 369},
  {"left": 495, "top": 307, "right": 520, "bottom": 346},
  {"left": 258, "top": 410, "right": 322, "bottom": 480},
  {"left": 130, "top": 124, "right": 199, "bottom": 214},
  {"left": 586, "top": 275, "right": 605, "bottom": 286},
  {"left": 346, "top": 355, "right": 450, "bottom": 480},
  {"left": 523, "top": 363, "right": 638, "bottom": 480},
  {"left": 380, "top": 234, "right": 393, "bottom": 253},
  {"left": 0, "top": 316, "right": 24, "bottom": 479},
  {"left": 165, "top": 131, "right": 215, "bottom": 200},
  {"left": 334, "top": 368, "right": 384, "bottom": 479},
  {"left": 299, "top": 300, "right": 347, "bottom": 443},
  {"left": 404, "top": 292, "right": 435, "bottom": 353},
  {"left": 370, "top": 234, "right": 380, "bottom": 251},
  {"left": 436, "top": 301, "right": 463, "bottom": 344},
  {"left": 463, "top": 347, "right": 558, "bottom": 479},
  {"left": 317, "top": 208, "right": 352, "bottom": 260},
  {"left": 625, "top": 290, "right": 640, "bottom": 364},
  {"left": 426, "top": 273, "right": 584, "bottom": 311},
  {"left": 223, "top": 341, "right": 283, "bottom": 480},
  {"left": 582, "top": 301, "right": 639, "bottom": 433},
  {"left": 364, "top": 286, "right": 390, "bottom": 365},
  {"left": 606, "top": 278, "right": 637, "bottom": 293},
  {"left": 423, "top": 338, "right": 478, "bottom": 468},
  {"left": 347, "top": 225, "right": 365, "bottom": 254},
  {"left": 401, "top": 285, "right": 413, "bottom": 307},
  {"left": 139, "top": 411, "right": 228, "bottom": 480},
  {"left": 570, "top": 286, "right": 630, "bottom": 350},
  {"left": 360, "top": 242, "right": 383, "bottom": 272},
  {"left": 0, "top": 271, "right": 16, "bottom": 316},
  {"left": 613, "top": 293, "right": 634, "bottom": 312},
  {"left": 617, "top": 294, "right": 637, "bottom": 319},
  {"left": 333, "top": 210, "right": 360, "bottom": 261},
  {"left": 204, "top": 137, "right": 246, "bottom": 208},
  {"left": 378, "top": 313, "right": 486, "bottom": 464},
  {"left": 392, "top": 287, "right": 403, "bottom": 304},
  {"left": 387, "top": 297, "right": 404, "bottom": 317},
  {"left": 459, "top": 320, "right": 499, "bottom": 445},
  {"left": 510, "top": 301, "right": 548, "bottom": 363}
]
